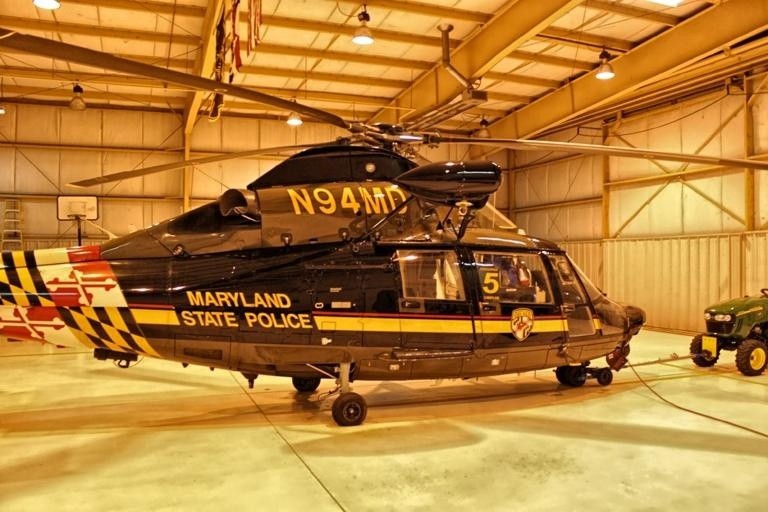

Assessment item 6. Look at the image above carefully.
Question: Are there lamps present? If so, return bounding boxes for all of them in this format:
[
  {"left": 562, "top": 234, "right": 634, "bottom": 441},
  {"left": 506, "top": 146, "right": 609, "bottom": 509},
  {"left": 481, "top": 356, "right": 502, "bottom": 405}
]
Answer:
[
  {"left": 473, "top": 113, "right": 491, "bottom": 139},
  {"left": 351, "top": 1, "right": 374, "bottom": 46},
  {"left": 286, "top": 97, "right": 304, "bottom": 127},
  {"left": 594, "top": 47, "right": 618, "bottom": 80},
  {"left": 67, "top": 83, "right": 87, "bottom": 113}
]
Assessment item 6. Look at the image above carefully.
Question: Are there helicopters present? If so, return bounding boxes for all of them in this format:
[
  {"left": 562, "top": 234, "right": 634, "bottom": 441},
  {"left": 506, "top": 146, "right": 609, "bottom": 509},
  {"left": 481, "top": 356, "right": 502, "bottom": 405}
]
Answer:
[{"left": 0, "top": 27, "right": 768, "bottom": 426}]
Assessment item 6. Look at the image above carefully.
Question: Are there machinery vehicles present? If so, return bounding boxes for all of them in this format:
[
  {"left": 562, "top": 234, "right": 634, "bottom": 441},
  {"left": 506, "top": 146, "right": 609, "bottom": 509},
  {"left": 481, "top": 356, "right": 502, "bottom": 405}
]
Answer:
[{"left": 690, "top": 286, "right": 768, "bottom": 376}]
